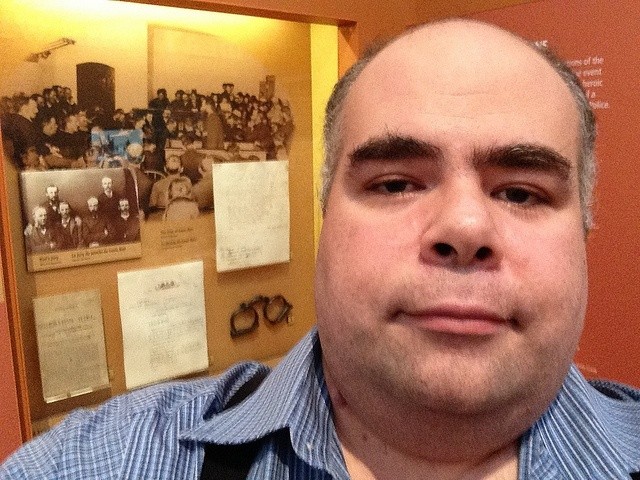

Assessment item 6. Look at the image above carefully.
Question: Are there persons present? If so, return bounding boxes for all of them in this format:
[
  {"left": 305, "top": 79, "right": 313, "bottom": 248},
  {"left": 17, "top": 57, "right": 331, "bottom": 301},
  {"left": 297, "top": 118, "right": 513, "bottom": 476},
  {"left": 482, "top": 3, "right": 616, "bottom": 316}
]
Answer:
[
  {"left": 2, "top": 70, "right": 294, "bottom": 256},
  {"left": 1, "top": 12, "right": 640, "bottom": 479}
]
